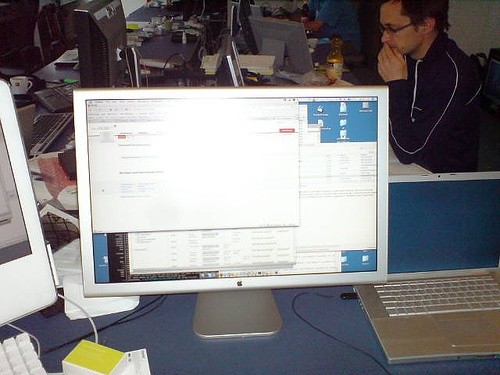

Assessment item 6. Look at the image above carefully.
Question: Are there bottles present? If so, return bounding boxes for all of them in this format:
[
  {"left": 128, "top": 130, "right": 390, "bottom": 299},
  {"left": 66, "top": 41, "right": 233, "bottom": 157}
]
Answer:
[
  {"left": 325, "top": 37, "right": 343, "bottom": 86},
  {"left": 300, "top": 3, "right": 309, "bottom": 24}
]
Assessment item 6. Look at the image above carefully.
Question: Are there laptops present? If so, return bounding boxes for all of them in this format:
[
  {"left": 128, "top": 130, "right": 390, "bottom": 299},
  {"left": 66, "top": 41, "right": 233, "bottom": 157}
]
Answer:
[{"left": 354, "top": 171, "right": 500, "bottom": 361}]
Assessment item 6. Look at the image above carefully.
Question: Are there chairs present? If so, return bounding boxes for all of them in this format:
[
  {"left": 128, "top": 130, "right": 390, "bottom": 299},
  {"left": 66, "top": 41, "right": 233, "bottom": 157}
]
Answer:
[{"left": 0, "top": 0, "right": 74, "bottom": 82}]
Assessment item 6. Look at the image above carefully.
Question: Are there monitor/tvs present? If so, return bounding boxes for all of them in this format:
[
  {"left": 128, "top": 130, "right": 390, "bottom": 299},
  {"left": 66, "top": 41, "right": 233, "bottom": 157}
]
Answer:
[
  {"left": 251, "top": 15, "right": 315, "bottom": 75},
  {"left": 74, "top": 0, "right": 140, "bottom": 87},
  {"left": 226, "top": 0, "right": 262, "bottom": 46},
  {"left": 72, "top": 88, "right": 386, "bottom": 342},
  {"left": 226, "top": 37, "right": 247, "bottom": 86},
  {"left": 0, "top": 80, "right": 58, "bottom": 331}
]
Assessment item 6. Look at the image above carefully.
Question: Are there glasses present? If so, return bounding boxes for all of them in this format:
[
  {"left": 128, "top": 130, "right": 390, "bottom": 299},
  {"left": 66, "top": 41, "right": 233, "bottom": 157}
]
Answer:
[{"left": 376, "top": 22, "right": 414, "bottom": 35}]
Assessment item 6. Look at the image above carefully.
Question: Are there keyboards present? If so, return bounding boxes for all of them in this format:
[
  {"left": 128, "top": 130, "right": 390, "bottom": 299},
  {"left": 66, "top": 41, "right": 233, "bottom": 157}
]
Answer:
[
  {"left": 29, "top": 113, "right": 72, "bottom": 157},
  {"left": 0, "top": 333, "right": 48, "bottom": 374},
  {"left": 55, "top": 49, "right": 80, "bottom": 68},
  {"left": 35, "top": 80, "right": 87, "bottom": 111}
]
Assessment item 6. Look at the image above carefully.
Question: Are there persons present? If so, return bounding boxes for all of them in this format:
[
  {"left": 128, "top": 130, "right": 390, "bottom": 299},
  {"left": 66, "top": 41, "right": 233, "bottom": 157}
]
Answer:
[
  {"left": 377, "top": 0, "right": 500, "bottom": 173},
  {"left": 264, "top": 0, "right": 364, "bottom": 69}
]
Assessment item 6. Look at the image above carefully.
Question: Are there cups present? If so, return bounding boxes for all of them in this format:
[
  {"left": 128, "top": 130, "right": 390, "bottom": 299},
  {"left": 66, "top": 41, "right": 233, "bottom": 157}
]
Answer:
[
  {"left": 9, "top": 75, "right": 33, "bottom": 95},
  {"left": 163, "top": 21, "right": 173, "bottom": 31}
]
